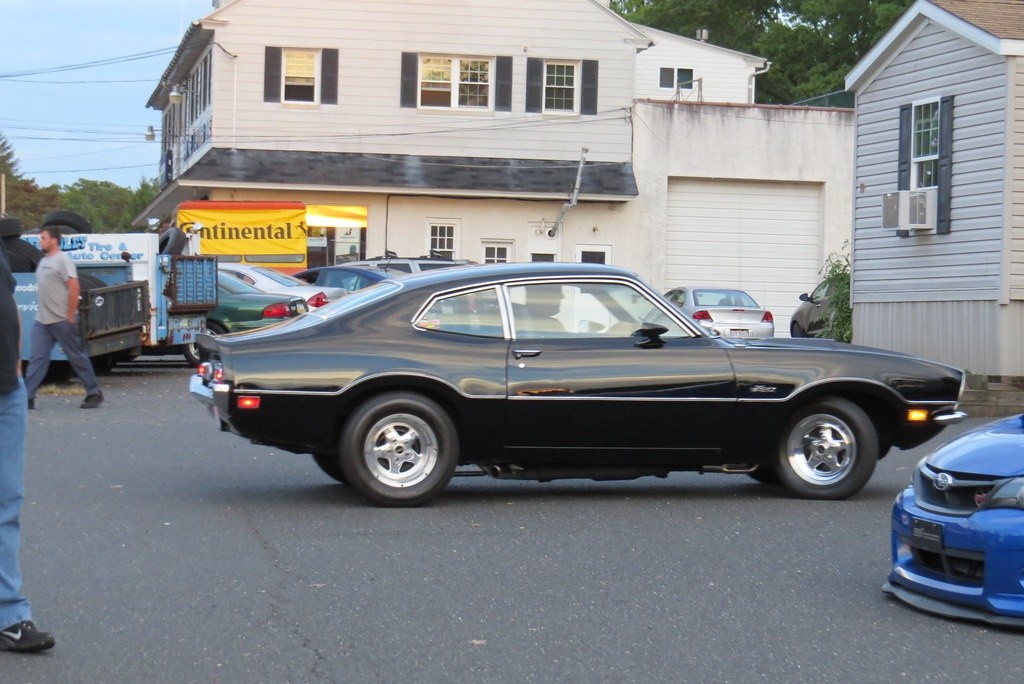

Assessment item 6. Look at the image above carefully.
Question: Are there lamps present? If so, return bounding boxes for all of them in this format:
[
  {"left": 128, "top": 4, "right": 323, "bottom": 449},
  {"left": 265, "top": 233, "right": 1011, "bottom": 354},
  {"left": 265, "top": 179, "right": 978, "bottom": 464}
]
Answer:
[
  {"left": 146, "top": 125, "right": 173, "bottom": 141},
  {"left": 169, "top": 82, "right": 200, "bottom": 103}
]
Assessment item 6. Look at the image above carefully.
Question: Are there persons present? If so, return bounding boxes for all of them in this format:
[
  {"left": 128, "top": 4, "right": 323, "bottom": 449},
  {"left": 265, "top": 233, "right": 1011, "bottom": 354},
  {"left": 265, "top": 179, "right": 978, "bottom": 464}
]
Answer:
[
  {"left": 24, "top": 225, "right": 107, "bottom": 410},
  {"left": 1, "top": 245, "right": 57, "bottom": 653},
  {"left": 523, "top": 284, "right": 565, "bottom": 332}
]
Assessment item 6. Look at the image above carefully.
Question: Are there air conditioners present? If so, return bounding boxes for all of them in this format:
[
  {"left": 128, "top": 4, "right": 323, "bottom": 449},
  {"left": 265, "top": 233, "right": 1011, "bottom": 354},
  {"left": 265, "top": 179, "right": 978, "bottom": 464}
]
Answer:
[{"left": 882, "top": 189, "right": 935, "bottom": 231}]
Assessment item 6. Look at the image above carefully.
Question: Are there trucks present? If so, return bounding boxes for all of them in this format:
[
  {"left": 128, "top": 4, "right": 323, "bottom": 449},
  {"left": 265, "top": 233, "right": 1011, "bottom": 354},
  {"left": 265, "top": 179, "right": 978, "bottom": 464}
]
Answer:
[{"left": 2, "top": 225, "right": 221, "bottom": 381}]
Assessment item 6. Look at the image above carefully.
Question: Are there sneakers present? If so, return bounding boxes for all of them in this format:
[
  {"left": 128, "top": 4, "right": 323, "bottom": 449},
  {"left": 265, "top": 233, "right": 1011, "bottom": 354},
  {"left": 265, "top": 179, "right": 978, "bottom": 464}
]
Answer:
[
  {"left": 1, "top": 621, "right": 56, "bottom": 652},
  {"left": 79, "top": 391, "right": 104, "bottom": 409},
  {"left": 28, "top": 399, "right": 34, "bottom": 410}
]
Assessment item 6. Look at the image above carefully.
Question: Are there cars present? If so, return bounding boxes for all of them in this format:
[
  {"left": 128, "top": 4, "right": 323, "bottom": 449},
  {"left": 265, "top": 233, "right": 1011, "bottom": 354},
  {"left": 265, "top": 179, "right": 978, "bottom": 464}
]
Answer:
[
  {"left": 663, "top": 286, "right": 775, "bottom": 341},
  {"left": 174, "top": 257, "right": 481, "bottom": 365},
  {"left": 190, "top": 259, "right": 969, "bottom": 512},
  {"left": 789, "top": 273, "right": 849, "bottom": 340},
  {"left": 883, "top": 411, "right": 1024, "bottom": 633}
]
